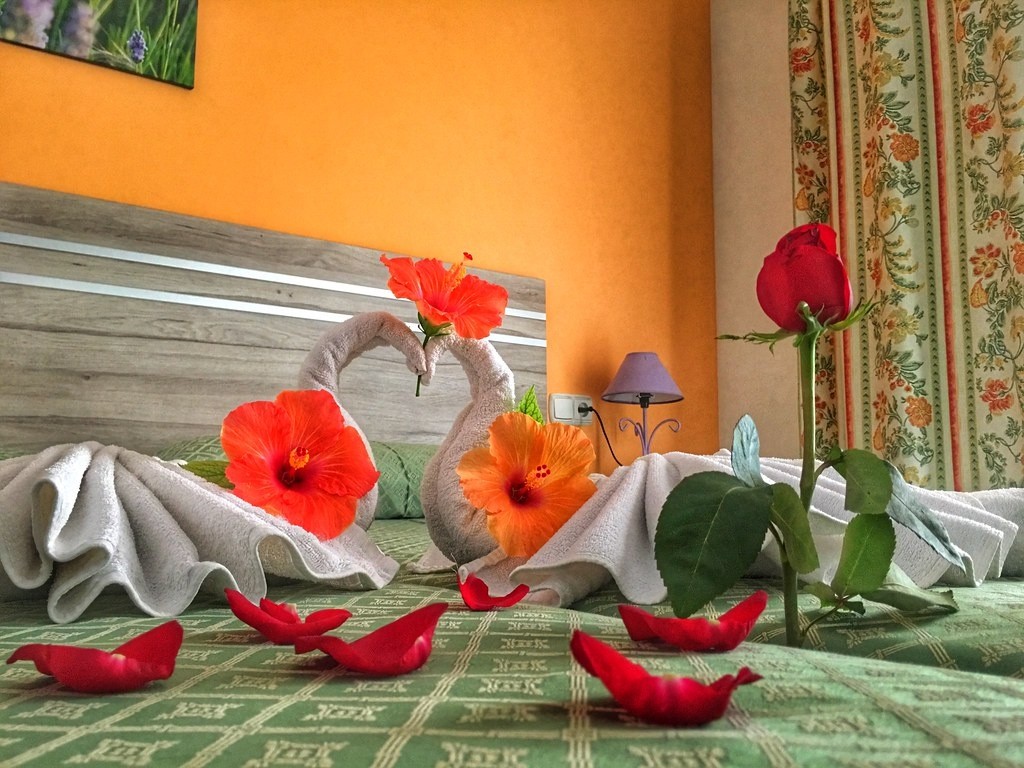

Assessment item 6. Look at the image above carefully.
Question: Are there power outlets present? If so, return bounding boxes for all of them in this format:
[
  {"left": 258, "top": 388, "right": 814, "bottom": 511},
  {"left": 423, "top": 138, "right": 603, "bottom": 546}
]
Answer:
[{"left": 573, "top": 395, "right": 593, "bottom": 426}]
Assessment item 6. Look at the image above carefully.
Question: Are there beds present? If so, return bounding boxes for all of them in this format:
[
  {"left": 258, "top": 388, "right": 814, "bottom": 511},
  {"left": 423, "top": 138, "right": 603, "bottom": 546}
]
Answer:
[{"left": 0, "top": 181, "right": 1024, "bottom": 768}]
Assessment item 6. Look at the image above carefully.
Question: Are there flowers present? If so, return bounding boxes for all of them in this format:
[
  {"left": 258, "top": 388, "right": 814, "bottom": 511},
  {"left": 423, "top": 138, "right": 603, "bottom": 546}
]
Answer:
[
  {"left": 217, "top": 252, "right": 599, "bottom": 562},
  {"left": 652, "top": 220, "right": 957, "bottom": 651}
]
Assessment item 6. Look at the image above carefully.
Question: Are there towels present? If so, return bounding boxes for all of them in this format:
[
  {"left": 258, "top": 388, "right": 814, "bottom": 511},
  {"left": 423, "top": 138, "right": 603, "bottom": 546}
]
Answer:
[
  {"left": 0, "top": 310, "right": 429, "bottom": 628},
  {"left": 421, "top": 317, "right": 1024, "bottom": 613}
]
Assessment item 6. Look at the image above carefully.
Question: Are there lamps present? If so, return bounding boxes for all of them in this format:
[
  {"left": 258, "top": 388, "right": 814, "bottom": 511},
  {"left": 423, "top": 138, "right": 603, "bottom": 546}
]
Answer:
[{"left": 601, "top": 350, "right": 684, "bottom": 457}]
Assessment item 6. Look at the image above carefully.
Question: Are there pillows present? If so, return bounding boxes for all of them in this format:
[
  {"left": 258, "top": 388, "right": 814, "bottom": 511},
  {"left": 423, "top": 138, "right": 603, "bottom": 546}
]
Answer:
[
  {"left": 153, "top": 434, "right": 229, "bottom": 463},
  {"left": 368, "top": 440, "right": 443, "bottom": 523}
]
